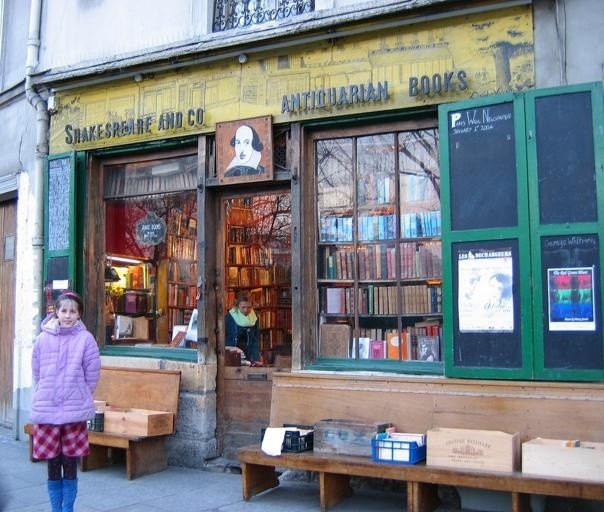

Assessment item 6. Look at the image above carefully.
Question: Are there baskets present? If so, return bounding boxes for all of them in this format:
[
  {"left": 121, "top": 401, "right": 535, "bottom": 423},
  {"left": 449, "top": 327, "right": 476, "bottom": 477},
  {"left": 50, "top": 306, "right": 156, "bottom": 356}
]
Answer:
[
  {"left": 371, "top": 433, "right": 426, "bottom": 464},
  {"left": 261, "top": 424, "right": 313, "bottom": 453}
]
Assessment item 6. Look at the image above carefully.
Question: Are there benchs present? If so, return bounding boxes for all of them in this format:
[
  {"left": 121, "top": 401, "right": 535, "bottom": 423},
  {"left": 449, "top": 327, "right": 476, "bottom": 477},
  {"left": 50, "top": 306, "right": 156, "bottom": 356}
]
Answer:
[
  {"left": 25, "top": 366, "right": 181, "bottom": 480},
  {"left": 236, "top": 373, "right": 604, "bottom": 512}
]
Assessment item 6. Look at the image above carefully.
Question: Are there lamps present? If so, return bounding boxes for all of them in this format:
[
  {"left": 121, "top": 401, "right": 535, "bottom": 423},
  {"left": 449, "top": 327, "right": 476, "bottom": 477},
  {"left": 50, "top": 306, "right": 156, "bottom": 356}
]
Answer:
[{"left": 46, "top": 95, "right": 60, "bottom": 114}]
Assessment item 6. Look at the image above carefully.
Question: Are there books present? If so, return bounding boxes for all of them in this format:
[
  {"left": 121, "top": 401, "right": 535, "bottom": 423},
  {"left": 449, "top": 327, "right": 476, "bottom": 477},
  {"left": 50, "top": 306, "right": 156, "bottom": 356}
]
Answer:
[
  {"left": 316, "top": 128, "right": 444, "bottom": 362},
  {"left": 256, "top": 305, "right": 291, "bottom": 368},
  {"left": 225, "top": 194, "right": 291, "bottom": 306},
  {"left": 166, "top": 192, "right": 197, "bottom": 349}
]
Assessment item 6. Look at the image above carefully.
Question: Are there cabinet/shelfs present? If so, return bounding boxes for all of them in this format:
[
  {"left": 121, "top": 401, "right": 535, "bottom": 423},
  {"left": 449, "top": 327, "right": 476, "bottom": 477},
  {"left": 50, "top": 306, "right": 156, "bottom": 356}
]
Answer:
[{"left": 105, "top": 137, "right": 442, "bottom": 367}]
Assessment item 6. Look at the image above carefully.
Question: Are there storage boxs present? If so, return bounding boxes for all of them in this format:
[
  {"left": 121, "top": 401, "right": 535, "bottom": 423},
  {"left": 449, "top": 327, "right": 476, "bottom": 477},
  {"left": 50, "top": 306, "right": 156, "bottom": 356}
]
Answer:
[
  {"left": 103, "top": 408, "right": 173, "bottom": 436},
  {"left": 426, "top": 428, "right": 520, "bottom": 472},
  {"left": 521, "top": 437, "right": 604, "bottom": 482},
  {"left": 313, "top": 419, "right": 392, "bottom": 458}
]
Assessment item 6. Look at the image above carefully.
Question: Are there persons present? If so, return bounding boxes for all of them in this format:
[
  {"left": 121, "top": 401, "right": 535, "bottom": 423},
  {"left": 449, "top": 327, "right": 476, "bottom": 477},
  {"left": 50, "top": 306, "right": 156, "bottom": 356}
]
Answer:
[
  {"left": 481, "top": 273, "right": 512, "bottom": 313},
  {"left": 224, "top": 289, "right": 263, "bottom": 367},
  {"left": 31, "top": 291, "right": 100, "bottom": 511},
  {"left": 219, "top": 123, "right": 267, "bottom": 177}
]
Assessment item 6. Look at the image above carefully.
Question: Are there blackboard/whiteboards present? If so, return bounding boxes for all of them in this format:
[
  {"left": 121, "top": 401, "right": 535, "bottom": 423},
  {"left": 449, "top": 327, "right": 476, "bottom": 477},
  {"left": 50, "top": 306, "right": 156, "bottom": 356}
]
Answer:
[
  {"left": 441, "top": 233, "right": 533, "bottom": 380},
  {"left": 524, "top": 81, "right": 603, "bottom": 230},
  {"left": 41, "top": 150, "right": 78, "bottom": 321},
  {"left": 530, "top": 232, "right": 603, "bottom": 380},
  {"left": 438, "top": 91, "right": 529, "bottom": 233}
]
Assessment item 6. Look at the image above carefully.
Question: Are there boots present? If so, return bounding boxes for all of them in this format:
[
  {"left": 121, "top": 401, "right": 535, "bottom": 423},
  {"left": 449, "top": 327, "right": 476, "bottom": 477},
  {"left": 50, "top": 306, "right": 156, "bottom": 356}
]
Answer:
[
  {"left": 47, "top": 478, "right": 63, "bottom": 512},
  {"left": 62, "top": 479, "right": 77, "bottom": 512}
]
